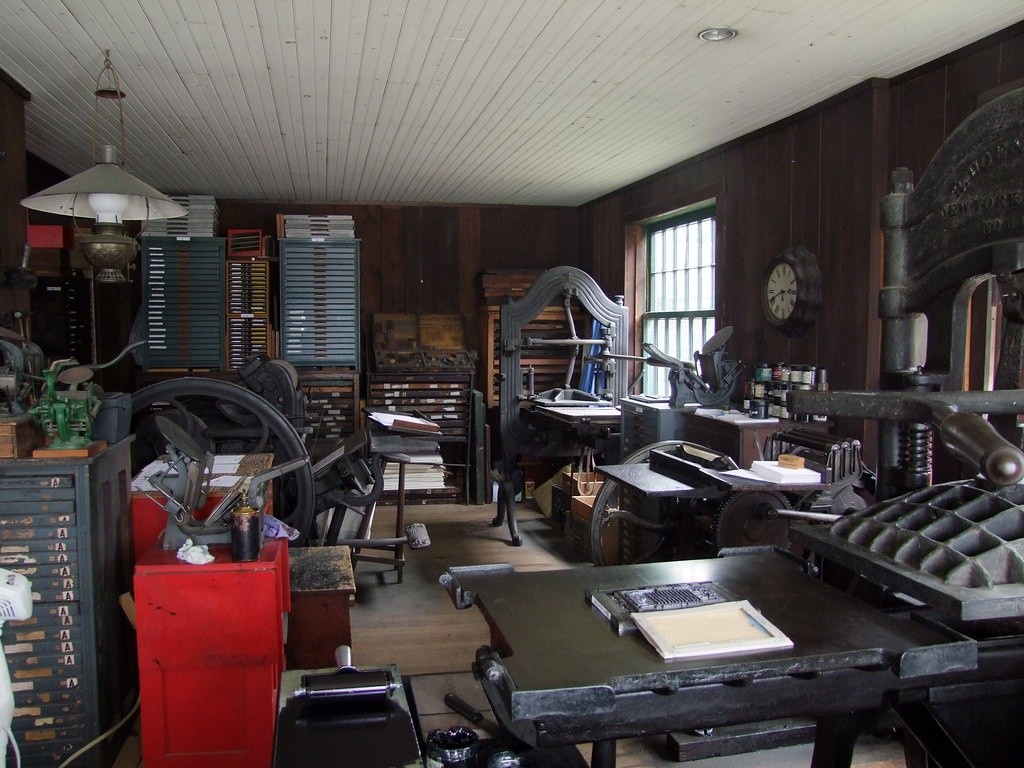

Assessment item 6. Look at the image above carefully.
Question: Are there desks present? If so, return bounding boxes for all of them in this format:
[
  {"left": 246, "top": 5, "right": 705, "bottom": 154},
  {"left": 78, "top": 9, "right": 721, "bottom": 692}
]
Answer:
[{"left": 287, "top": 546, "right": 357, "bottom": 672}]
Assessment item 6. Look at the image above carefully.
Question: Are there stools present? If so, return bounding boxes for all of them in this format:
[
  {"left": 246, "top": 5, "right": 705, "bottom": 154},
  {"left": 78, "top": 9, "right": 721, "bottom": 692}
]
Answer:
[{"left": 325, "top": 452, "right": 409, "bottom": 584}]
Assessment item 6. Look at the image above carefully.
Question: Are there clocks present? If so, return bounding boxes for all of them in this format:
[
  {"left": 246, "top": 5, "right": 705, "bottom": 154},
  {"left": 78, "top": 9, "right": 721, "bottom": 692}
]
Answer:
[{"left": 760, "top": 241, "right": 823, "bottom": 339}]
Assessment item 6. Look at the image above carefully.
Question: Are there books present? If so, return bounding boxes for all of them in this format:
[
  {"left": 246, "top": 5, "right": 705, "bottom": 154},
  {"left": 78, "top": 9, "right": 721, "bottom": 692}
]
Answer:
[
  {"left": 382, "top": 462, "right": 446, "bottom": 490},
  {"left": 749, "top": 460, "right": 821, "bottom": 484}
]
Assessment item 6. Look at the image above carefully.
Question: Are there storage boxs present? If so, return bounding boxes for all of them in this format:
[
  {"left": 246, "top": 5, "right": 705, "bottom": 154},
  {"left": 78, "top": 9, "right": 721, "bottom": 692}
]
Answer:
[
  {"left": 371, "top": 311, "right": 477, "bottom": 374},
  {"left": 93, "top": 392, "right": 132, "bottom": 445},
  {"left": 529, "top": 463, "right": 619, "bottom": 562},
  {"left": 0, "top": 412, "right": 44, "bottom": 460}
]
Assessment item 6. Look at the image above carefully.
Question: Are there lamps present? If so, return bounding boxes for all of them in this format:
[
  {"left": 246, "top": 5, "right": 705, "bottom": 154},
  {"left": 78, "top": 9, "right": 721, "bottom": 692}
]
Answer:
[{"left": 20, "top": 49, "right": 188, "bottom": 282}]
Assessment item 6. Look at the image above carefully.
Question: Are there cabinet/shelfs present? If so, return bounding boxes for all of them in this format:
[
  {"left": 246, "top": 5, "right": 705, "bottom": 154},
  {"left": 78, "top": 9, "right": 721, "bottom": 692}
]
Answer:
[
  {"left": 619, "top": 394, "right": 835, "bottom": 565},
  {"left": 141, "top": 194, "right": 593, "bottom": 507}
]
